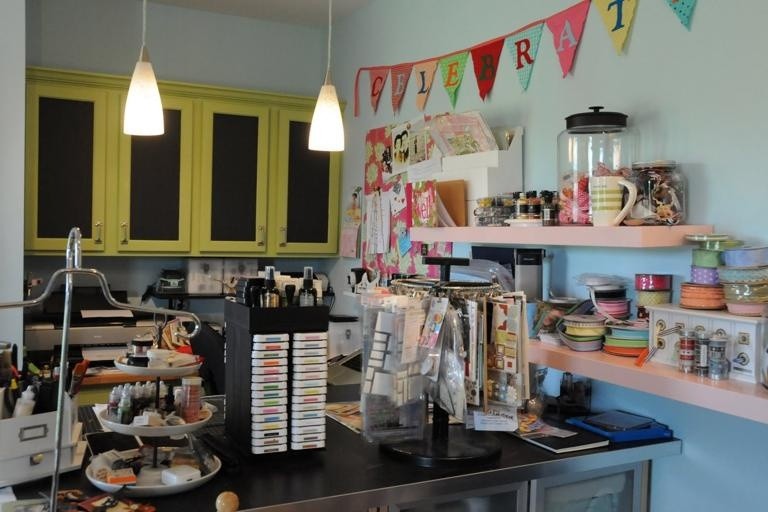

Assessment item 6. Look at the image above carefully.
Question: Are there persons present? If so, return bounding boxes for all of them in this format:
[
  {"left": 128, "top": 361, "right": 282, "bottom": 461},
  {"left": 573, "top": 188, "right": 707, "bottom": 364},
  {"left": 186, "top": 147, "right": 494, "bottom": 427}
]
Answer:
[
  {"left": 392, "top": 134, "right": 406, "bottom": 162},
  {"left": 343, "top": 192, "right": 361, "bottom": 227},
  {"left": 399, "top": 130, "right": 411, "bottom": 161}
]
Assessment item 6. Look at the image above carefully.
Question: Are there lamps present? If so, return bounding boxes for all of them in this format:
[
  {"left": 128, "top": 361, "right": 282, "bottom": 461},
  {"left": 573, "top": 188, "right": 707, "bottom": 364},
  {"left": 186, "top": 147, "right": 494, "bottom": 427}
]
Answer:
[
  {"left": 307, "top": 0, "right": 345, "bottom": 153},
  {"left": 122, "top": 0, "right": 165, "bottom": 137}
]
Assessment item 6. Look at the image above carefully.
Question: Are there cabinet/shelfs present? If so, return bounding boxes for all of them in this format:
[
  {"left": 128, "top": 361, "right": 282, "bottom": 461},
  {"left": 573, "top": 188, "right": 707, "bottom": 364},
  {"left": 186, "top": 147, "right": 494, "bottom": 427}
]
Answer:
[
  {"left": 24, "top": 76, "right": 198, "bottom": 257},
  {"left": 409, "top": 222, "right": 768, "bottom": 424},
  {"left": 198, "top": 96, "right": 340, "bottom": 256}
]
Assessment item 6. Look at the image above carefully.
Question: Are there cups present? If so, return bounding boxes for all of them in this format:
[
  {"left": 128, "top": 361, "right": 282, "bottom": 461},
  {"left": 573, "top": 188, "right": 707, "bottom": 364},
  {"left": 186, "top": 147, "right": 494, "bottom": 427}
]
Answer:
[{"left": 590, "top": 175, "right": 638, "bottom": 227}]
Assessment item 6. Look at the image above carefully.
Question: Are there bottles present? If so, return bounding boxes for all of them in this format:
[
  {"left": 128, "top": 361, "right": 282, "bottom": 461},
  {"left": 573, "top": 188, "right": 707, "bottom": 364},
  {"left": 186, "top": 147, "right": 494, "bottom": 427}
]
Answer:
[
  {"left": 513, "top": 190, "right": 557, "bottom": 225},
  {"left": 8, "top": 378, "right": 36, "bottom": 417},
  {"left": 180, "top": 375, "right": 203, "bottom": 421},
  {"left": 259, "top": 266, "right": 281, "bottom": 308},
  {"left": 556, "top": 105, "right": 631, "bottom": 226},
  {"left": 297, "top": 267, "right": 317, "bottom": 308},
  {"left": 678, "top": 328, "right": 727, "bottom": 381},
  {"left": 107, "top": 381, "right": 168, "bottom": 424},
  {"left": 624, "top": 160, "right": 687, "bottom": 225}
]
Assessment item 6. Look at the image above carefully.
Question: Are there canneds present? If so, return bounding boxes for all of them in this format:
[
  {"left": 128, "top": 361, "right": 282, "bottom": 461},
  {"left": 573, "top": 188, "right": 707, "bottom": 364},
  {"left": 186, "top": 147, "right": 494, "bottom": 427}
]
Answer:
[{"left": 626, "top": 161, "right": 686, "bottom": 225}]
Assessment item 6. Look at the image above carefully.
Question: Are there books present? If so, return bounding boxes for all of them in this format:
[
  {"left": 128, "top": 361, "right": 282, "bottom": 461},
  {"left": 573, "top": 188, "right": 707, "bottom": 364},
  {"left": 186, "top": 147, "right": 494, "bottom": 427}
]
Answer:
[{"left": 509, "top": 415, "right": 610, "bottom": 454}]
